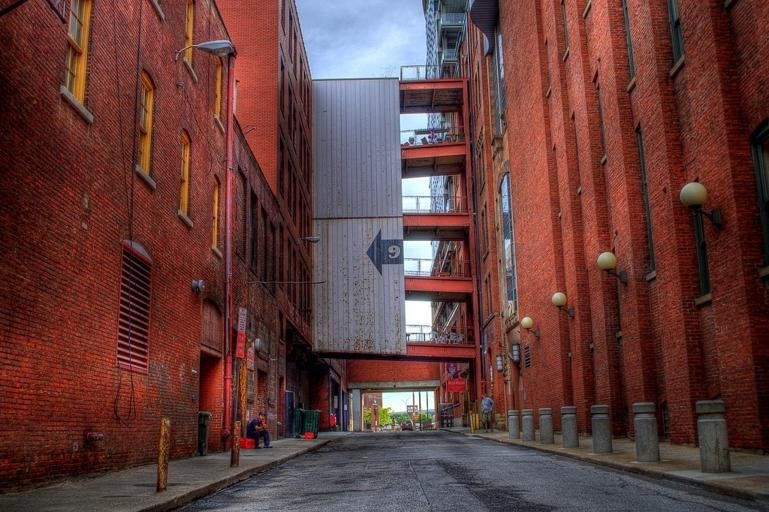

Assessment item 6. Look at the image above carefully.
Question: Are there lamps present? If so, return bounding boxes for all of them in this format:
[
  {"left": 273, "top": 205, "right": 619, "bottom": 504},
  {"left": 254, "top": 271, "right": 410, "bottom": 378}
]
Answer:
[
  {"left": 597, "top": 252, "right": 628, "bottom": 287},
  {"left": 678, "top": 180, "right": 724, "bottom": 228},
  {"left": 301, "top": 236, "right": 321, "bottom": 244},
  {"left": 521, "top": 290, "right": 574, "bottom": 338},
  {"left": 511, "top": 343, "right": 521, "bottom": 362},
  {"left": 175, "top": 39, "right": 235, "bottom": 61},
  {"left": 494, "top": 353, "right": 506, "bottom": 372}
]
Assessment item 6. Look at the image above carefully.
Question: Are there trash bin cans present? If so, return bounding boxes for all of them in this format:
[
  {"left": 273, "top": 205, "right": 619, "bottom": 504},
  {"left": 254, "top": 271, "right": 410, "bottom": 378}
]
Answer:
[
  {"left": 200, "top": 414, "right": 209, "bottom": 458},
  {"left": 298, "top": 411, "right": 321, "bottom": 433}
]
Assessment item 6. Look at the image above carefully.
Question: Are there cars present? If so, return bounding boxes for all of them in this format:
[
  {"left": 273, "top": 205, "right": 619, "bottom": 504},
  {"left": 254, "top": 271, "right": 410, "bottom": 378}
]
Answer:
[{"left": 401, "top": 419, "right": 414, "bottom": 431}]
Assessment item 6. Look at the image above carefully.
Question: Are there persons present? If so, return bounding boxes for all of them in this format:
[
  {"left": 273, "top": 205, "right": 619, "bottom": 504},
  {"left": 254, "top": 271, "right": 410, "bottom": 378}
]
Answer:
[
  {"left": 246, "top": 412, "right": 272, "bottom": 449},
  {"left": 480, "top": 392, "right": 494, "bottom": 432}
]
